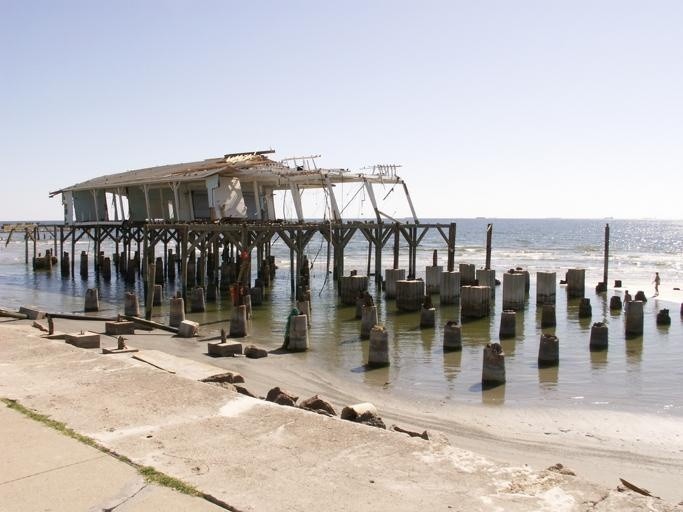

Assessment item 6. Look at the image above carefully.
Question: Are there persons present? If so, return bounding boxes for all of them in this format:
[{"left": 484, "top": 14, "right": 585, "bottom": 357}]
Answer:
[
  {"left": 654, "top": 272, "right": 660, "bottom": 292},
  {"left": 623, "top": 290, "right": 631, "bottom": 303}
]
